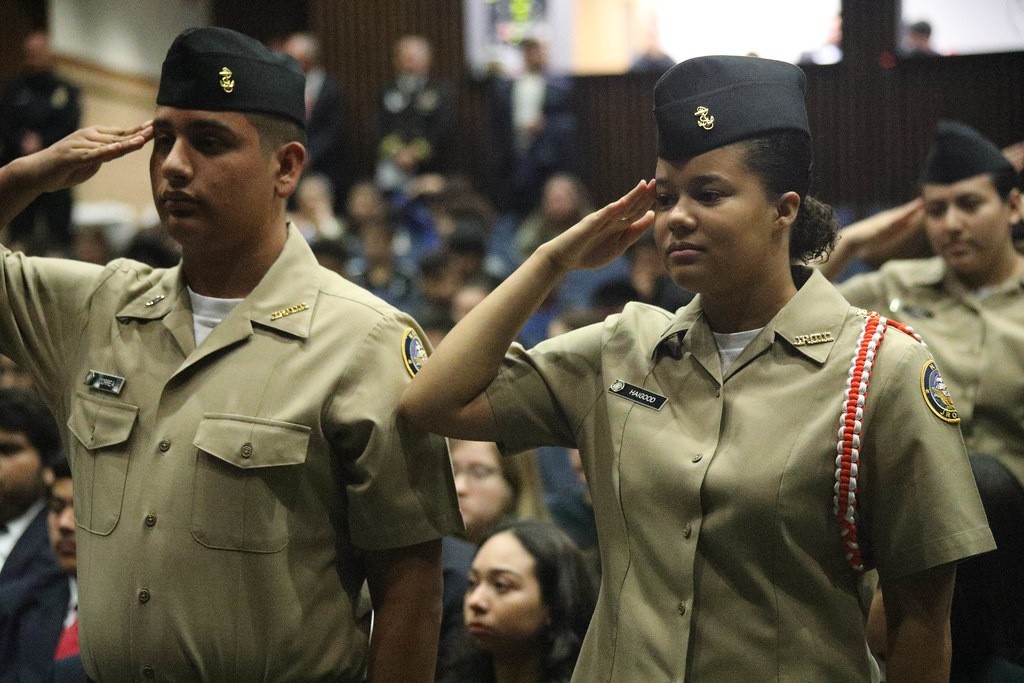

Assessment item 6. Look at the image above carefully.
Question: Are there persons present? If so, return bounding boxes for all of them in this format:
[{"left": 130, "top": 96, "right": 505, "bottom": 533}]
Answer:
[
  {"left": 911, "top": 22, "right": 936, "bottom": 55},
  {"left": 796, "top": 121, "right": 1024, "bottom": 482},
  {"left": 0, "top": 28, "right": 467, "bottom": 683},
  {"left": 449, "top": 36, "right": 696, "bottom": 683},
  {"left": 398, "top": 55, "right": 998, "bottom": 683}
]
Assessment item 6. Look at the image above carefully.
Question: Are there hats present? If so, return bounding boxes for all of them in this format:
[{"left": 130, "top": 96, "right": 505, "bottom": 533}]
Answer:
[
  {"left": 156, "top": 27, "right": 309, "bottom": 125},
  {"left": 915, "top": 121, "right": 1020, "bottom": 187},
  {"left": 654, "top": 53, "right": 817, "bottom": 161}
]
